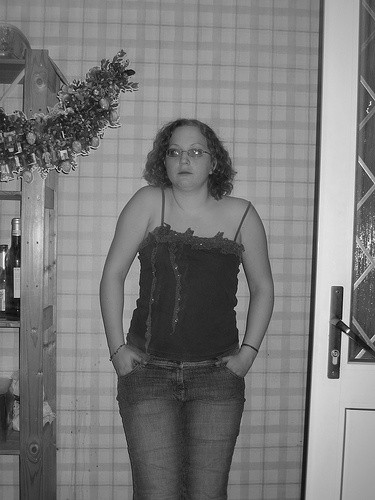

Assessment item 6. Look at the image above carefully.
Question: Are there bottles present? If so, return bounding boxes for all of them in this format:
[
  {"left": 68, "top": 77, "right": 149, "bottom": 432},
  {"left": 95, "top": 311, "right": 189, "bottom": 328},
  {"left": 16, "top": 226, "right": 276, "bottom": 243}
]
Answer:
[
  {"left": 5, "top": 217, "right": 21, "bottom": 322},
  {"left": 0, "top": 244, "right": 8, "bottom": 318}
]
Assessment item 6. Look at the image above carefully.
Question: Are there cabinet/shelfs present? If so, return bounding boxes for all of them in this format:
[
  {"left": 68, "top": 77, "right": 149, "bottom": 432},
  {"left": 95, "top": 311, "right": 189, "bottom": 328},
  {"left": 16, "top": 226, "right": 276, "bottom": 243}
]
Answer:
[{"left": 0, "top": 50, "right": 68, "bottom": 500}]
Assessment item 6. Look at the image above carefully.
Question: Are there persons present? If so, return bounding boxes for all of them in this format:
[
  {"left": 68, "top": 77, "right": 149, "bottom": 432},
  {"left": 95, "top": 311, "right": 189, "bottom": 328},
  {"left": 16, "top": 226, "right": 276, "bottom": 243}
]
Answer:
[{"left": 99, "top": 118, "right": 274, "bottom": 500}]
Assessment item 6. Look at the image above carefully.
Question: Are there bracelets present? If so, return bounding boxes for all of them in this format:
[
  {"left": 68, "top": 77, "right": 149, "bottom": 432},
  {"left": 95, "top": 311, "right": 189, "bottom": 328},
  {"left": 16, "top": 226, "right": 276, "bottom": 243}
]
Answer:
[
  {"left": 109, "top": 344, "right": 125, "bottom": 361},
  {"left": 241, "top": 344, "right": 258, "bottom": 353}
]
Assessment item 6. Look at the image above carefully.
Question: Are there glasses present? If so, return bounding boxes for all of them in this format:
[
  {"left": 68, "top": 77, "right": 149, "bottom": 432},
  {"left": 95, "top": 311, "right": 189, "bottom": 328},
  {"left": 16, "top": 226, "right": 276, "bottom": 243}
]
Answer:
[{"left": 166, "top": 148, "right": 211, "bottom": 158}]
